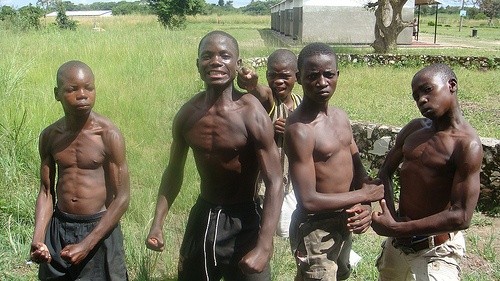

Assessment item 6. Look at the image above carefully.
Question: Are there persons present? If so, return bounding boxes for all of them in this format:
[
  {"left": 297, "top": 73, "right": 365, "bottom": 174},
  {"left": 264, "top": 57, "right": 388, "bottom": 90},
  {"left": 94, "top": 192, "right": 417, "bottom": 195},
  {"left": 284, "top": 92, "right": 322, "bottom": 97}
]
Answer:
[
  {"left": 283, "top": 42, "right": 385, "bottom": 281},
  {"left": 29, "top": 60, "right": 130, "bottom": 281},
  {"left": 236, "top": 49, "right": 361, "bottom": 267},
  {"left": 370, "top": 62, "right": 484, "bottom": 281},
  {"left": 145, "top": 31, "right": 284, "bottom": 281}
]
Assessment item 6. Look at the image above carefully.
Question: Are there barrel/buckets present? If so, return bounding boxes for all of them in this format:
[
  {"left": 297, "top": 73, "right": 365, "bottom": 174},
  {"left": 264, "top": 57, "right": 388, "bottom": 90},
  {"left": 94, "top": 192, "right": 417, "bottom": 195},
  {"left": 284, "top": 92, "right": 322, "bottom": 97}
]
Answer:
[{"left": 472, "top": 30, "right": 477, "bottom": 37}]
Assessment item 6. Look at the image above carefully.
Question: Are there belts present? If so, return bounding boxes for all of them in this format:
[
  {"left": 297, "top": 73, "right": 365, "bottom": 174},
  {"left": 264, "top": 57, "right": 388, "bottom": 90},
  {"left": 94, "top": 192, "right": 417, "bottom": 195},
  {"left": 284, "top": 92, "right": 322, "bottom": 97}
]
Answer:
[{"left": 392, "top": 231, "right": 459, "bottom": 256}]
